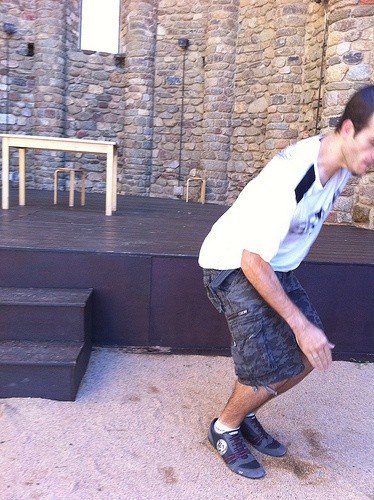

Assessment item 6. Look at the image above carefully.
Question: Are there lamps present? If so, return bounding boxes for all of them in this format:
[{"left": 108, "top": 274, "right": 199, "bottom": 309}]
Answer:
[
  {"left": 178, "top": 38, "right": 189, "bottom": 49},
  {"left": 27, "top": 43, "right": 35, "bottom": 58},
  {"left": 3, "top": 22, "right": 15, "bottom": 34},
  {"left": 114, "top": 53, "right": 126, "bottom": 68}
]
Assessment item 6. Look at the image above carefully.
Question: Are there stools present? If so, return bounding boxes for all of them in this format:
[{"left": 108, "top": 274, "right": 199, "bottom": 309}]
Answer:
[
  {"left": 54, "top": 167, "right": 85, "bottom": 208},
  {"left": 185, "top": 175, "right": 207, "bottom": 205}
]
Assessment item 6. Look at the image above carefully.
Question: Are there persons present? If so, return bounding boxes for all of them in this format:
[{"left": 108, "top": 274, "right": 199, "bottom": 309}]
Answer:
[{"left": 198, "top": 84, "right": 374, "bottom": 481}]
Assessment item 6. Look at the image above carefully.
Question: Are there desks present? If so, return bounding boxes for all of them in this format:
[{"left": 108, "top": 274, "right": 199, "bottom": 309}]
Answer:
[{"left": 0, "top": 133, "right": 120, "bottom": 216}]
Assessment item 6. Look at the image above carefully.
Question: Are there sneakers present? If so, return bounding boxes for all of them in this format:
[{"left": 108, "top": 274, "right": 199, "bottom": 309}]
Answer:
[
  {"left": 207, "top": 417, "right": 266, "bottom": 479},
  {"left": 240, "top": 413, "right": 288, "bottom": 457}
]
